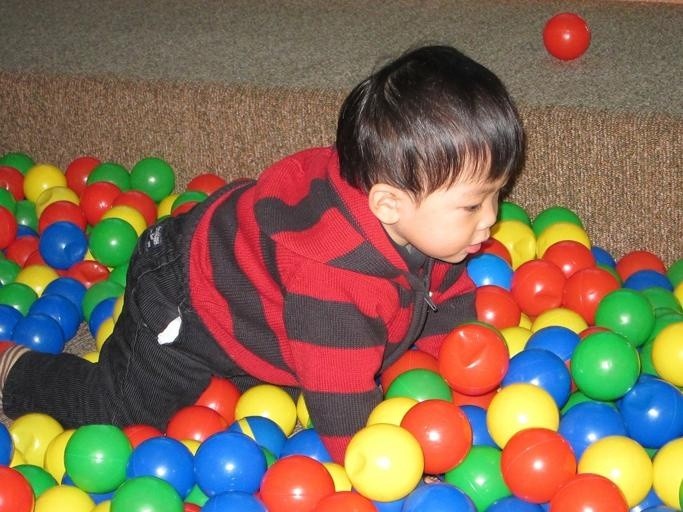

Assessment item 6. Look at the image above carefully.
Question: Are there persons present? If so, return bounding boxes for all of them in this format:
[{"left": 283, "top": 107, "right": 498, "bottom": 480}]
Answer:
[{"left": 0, "top": 45, "right": 528, "bottom": 463}]
